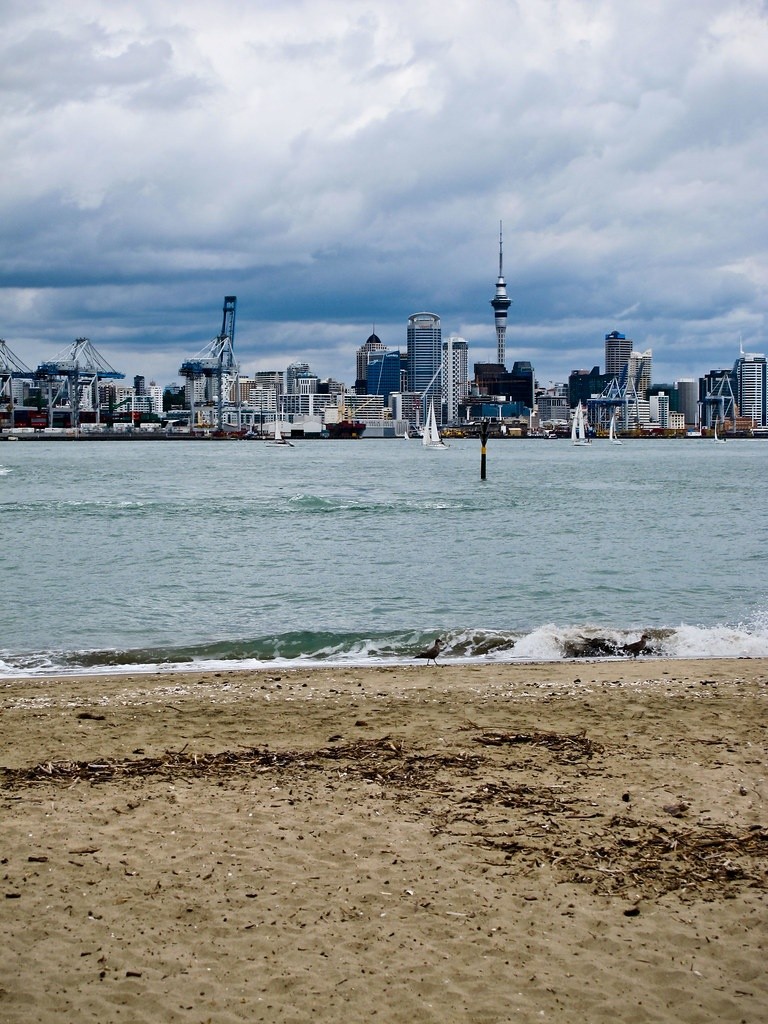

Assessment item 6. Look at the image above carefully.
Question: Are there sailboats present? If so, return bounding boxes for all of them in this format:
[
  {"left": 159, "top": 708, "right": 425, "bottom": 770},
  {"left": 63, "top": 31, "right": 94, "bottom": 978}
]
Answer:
[
  {"left": 270, "top": 412, "right": 288, "bottom": 444},
  {"left": 610, "top": 414, "right": 623, "bottom": 446},
  {"left": 710, "top": 414, "right": 726, "bottom": 443},
  {"left": 421, "top": 396, "right": 450, "bottom": 450},
  {"left": 570, "top": 399, "right": 593, "bottom": 447},
  {"left": 404, "top": 430, "right": 409, "bottom": 440}
]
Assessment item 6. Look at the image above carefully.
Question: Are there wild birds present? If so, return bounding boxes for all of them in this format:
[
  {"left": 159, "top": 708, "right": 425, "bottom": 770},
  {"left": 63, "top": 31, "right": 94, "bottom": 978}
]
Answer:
[
  {"left": 414, "top": 638, "right": 443, "bottom": 667},
  {"left": 619, "top": 635, "right": 650, "bottom": 658}
]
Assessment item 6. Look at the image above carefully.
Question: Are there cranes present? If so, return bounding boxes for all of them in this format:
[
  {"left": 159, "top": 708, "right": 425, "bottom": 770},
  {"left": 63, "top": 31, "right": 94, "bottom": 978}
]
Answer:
[
  {"left": 0, "top": 334, "right": 126, "bottom": 430},
  {"left": 178, "top": 295, "right": 244, "bottom": 433}
]
